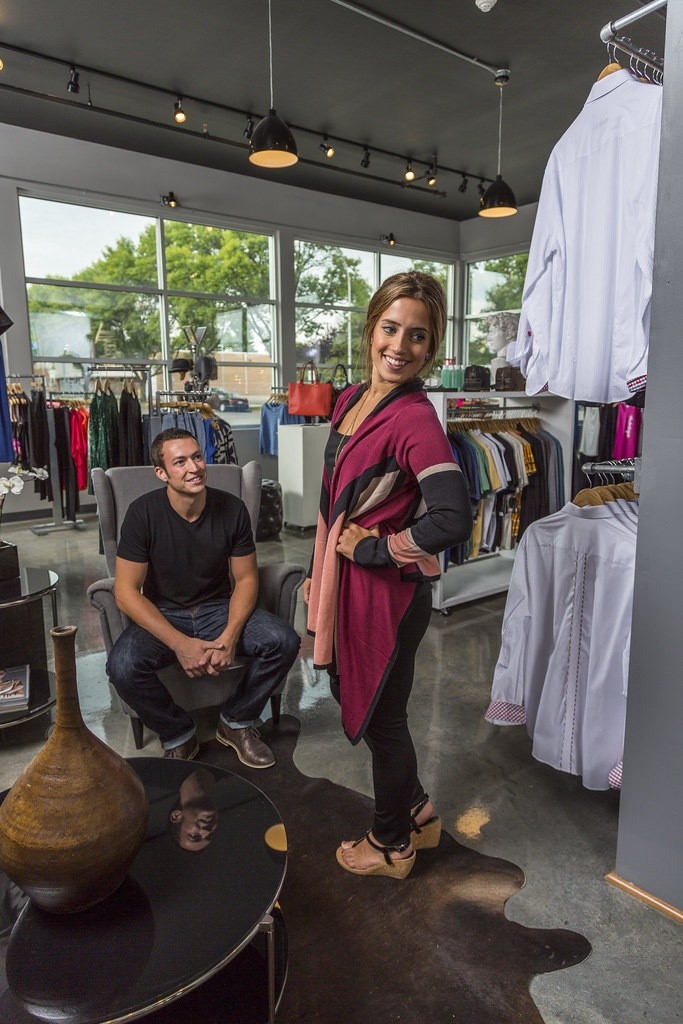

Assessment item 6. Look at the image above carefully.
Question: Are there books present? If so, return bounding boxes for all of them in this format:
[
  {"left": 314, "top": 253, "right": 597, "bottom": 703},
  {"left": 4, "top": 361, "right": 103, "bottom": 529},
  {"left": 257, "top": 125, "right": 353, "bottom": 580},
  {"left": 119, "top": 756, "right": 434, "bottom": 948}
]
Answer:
[{"left": 0, "top": 664, "right": 30, "bottom": 711}]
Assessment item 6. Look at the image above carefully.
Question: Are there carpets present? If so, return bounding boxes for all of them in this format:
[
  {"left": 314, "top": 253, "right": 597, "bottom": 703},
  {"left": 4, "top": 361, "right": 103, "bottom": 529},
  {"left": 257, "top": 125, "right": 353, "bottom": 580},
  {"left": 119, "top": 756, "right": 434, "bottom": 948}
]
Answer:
[{"left": 143, "top": 714, "right": 590, "bottom": 1023}]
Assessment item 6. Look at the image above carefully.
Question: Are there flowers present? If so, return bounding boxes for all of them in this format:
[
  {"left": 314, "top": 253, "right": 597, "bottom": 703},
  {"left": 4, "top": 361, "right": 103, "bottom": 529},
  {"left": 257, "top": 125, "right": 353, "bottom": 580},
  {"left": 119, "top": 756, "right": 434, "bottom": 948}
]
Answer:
[{"left": 0, "top": 461, "right": 49, "bottom": 513}]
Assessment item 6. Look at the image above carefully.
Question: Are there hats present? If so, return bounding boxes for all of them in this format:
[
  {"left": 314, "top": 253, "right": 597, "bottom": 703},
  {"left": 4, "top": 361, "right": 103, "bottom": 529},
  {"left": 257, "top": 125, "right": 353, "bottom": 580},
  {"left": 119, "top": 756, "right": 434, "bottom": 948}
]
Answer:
[
  {"left": 169, "top": 359, "right": 192, "bottom": 372},
  {"left": 192, "top": 355, "right": 218, "bottom": 381}
]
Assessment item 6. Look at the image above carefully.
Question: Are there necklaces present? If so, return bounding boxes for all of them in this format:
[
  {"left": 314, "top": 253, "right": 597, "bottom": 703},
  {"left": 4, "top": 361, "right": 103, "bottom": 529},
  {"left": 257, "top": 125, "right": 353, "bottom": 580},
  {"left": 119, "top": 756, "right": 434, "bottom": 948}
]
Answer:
[{"left": 335, "top": 386, "right": 372, "bottom": 466}]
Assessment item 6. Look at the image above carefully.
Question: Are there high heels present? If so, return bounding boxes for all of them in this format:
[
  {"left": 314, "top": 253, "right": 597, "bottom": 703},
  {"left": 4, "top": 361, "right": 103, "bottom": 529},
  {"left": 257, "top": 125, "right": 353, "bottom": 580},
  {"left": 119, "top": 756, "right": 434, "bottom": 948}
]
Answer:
[
  {"left": 411, "top": 793, "right": 441, "bottom": 850},
  {"left": 337, "top": 828, "right": 417, "bottom": 879}
]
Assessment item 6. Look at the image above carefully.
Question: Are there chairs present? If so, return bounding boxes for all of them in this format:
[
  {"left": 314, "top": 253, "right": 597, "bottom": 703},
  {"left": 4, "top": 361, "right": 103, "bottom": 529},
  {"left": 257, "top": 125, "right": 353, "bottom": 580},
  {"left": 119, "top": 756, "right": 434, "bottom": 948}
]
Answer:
[{"left": 88, "top": 459, "right": 310, "bottom": 746}]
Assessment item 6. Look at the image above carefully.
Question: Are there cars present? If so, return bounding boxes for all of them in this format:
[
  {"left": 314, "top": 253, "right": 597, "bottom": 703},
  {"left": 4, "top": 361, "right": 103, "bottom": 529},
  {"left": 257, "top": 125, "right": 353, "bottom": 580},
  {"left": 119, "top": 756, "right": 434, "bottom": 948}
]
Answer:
[{"left": 205, "top": 386, "right": 249, "bottom": 413}]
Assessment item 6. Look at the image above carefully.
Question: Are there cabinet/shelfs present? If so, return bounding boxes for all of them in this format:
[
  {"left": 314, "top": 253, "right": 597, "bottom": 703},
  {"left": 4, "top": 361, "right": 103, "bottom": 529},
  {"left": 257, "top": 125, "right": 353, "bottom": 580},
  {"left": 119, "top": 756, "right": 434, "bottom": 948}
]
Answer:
[{"left": 1, "top": 568, "right": 62, "bottom": 731}]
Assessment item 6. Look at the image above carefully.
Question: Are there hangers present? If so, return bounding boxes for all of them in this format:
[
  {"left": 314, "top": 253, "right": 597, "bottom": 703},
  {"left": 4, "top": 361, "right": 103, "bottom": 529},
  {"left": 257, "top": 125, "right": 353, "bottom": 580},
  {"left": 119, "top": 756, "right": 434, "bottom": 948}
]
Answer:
[
  {"left": 268, "top": 385, "right": 289, "bottom": 406},
  {"left": 570, "top": 453, "right": 642, "bottom": 508},
  {"left": 438, "top": 403, "right": 544, "bottom": 438},
  {"left": 595, "top": 31, "right": 664, "bottom": 87},
  {"left": 5, "top": 362, "right": 220, "bottom": 429}
]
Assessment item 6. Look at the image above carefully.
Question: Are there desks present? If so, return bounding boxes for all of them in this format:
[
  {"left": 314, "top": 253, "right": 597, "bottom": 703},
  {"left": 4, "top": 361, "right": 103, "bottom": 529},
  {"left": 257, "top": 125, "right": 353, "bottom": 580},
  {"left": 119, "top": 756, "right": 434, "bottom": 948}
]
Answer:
[{"left": 1, "top": 757, "right": 290, "bottom": 1024}]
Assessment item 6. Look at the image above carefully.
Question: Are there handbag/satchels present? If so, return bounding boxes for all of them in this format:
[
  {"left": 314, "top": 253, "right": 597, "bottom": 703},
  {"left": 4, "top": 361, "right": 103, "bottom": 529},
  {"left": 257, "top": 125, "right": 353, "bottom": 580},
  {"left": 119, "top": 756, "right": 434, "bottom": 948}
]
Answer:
[
  {"left": 495, "top": 362, "right": 523, "bottom": 391},
  {"left": 289, "top": 360, "right": 332, "bottom": 416},
  {"left": 441, "top": 363, "right": 464, "bottom": 387},
  {"left": 462, "top": 365, "right": 491, "bottom": 391},
  {"left": 322, "top": 365, "right": 352, "bottom": 420}
]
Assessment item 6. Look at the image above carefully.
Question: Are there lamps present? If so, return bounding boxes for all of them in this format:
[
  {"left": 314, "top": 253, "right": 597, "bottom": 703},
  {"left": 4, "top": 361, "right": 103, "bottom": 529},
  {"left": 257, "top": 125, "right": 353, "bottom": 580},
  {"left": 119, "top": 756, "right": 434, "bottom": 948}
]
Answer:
[
  {"left": 403, "top": 159, "right": 487, "bottom": 194},
  {"left": 386, "top": 233, "right": 395, "bottom": 246},
  {"left": 479, "top": 76, "right": 518, "bottom": 218},
  {"left": 67, "top": 70, "right": 79, "bottom": 94},
  {"left": 242, "top": 116, "right": 258, "bottom": 137},
  {"left": 360, "top": 147, "right": 370, "bottom": 167},
  {"left": 173, "top": 97, "right": 187, "bottom": 123},
  {"left": 161, "top": 191, "right": 177, "bottom": 207},
  {"left": 250, "top": 0, "right": 299, "bottom": 168},
  {"left": 319, "top": 138, "right": 335, "bottom": 157}
]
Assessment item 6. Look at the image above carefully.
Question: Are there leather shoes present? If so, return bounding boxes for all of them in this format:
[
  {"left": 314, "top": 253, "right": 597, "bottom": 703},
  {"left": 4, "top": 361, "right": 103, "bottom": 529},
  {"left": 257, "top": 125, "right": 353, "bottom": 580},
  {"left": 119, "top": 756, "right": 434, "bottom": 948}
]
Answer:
[
  {"left": 216, "top": 717, "right": 276, "bottom": 768},
  {"left": 162, "top": 734, "right": 201, "bottom": 760}
]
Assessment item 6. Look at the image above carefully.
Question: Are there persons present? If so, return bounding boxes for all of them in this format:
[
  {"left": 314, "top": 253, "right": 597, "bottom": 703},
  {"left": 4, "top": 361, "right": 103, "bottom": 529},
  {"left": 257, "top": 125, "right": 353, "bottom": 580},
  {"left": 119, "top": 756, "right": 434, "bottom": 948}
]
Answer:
[
  {"left": 0, "top": 668, "right": 24, "bottom": 698},
  {"left": 303, "top": 270, "right": 474, "bottom": 875},
  {"left": 106, "top": 429, "right": 301, "bottom": 769},
  {"left": 153, "top": 769, "right": 232, "bottom": 854}
]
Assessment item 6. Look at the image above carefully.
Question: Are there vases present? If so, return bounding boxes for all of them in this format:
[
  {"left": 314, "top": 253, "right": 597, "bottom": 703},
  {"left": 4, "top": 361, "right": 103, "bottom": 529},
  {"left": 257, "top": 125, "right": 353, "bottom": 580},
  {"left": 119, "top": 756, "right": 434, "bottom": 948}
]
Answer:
[{"left": 1, "top": 624, "right": 147, "bottom": 914}]
